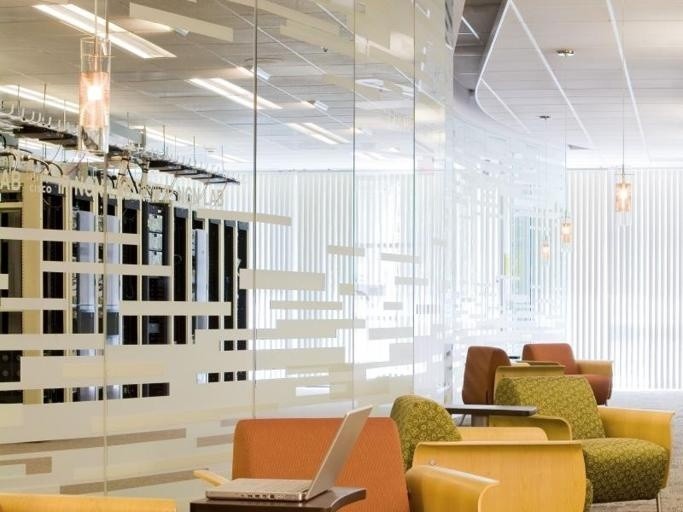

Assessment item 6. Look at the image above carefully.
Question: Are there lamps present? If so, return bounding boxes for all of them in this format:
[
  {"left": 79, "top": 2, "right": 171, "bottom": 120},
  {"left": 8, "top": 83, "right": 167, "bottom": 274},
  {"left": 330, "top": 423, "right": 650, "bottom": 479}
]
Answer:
[
  {"left": 539, "top": 0, "right": 632, "bottom": 261},
  {"left": 77, "top": 0, "right": 111, "bottom": 154}
]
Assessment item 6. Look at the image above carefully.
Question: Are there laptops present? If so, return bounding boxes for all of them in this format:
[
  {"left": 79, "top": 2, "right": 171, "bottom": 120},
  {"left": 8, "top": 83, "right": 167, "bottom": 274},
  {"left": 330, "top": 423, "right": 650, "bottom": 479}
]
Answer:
[{"left": 206, "top": 404, "right": 375, "bottom": 502}]
